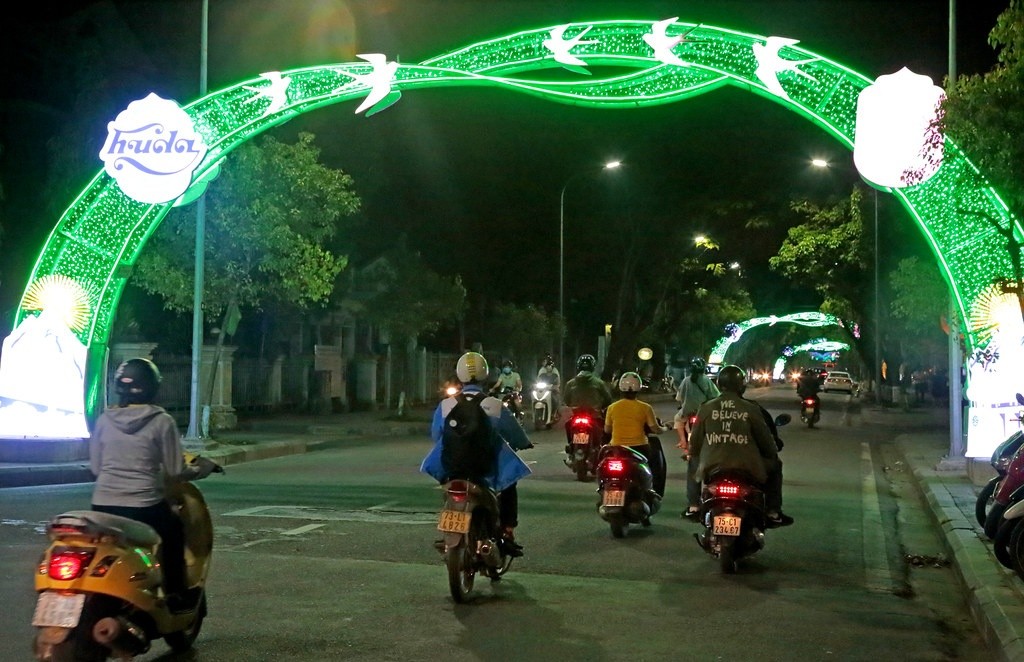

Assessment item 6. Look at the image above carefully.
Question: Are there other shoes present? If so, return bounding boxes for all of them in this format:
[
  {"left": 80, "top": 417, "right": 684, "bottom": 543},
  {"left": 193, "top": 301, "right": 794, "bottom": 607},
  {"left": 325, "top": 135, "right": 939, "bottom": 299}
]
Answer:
[
  {"left": 501, "top": 529, "right": 525, "bottom": 558},
  {"left": 674, "top": 440, "right": 687, "bottom": 449},
  {"left": 434, "top": 539, "right": 446, "bottom": 549},
  {"left": 565, "top": 457, "right": 574, "bottom": 464}
]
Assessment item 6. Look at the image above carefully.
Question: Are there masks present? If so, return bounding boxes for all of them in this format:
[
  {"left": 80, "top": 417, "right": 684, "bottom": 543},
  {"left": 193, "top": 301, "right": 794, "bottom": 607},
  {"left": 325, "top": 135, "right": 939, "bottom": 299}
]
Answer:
[{"left": 503, "top": 368, "right": 512, "bottom": 373}]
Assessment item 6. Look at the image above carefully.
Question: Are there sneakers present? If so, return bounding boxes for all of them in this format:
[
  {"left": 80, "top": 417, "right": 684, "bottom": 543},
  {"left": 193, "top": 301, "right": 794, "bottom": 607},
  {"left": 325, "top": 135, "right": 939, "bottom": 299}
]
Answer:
[
  {"left": 765, "top": 512, "right": 793, "bottom": 530},
  {"left": 681, "top": 507, "right": 701, "bottom": 523}
]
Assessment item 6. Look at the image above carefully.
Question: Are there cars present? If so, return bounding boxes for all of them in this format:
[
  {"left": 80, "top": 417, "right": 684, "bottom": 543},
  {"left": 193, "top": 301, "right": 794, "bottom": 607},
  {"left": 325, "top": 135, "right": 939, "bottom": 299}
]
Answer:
[{"left": 824, "top": 371, "right": 854, "bottom": 396}]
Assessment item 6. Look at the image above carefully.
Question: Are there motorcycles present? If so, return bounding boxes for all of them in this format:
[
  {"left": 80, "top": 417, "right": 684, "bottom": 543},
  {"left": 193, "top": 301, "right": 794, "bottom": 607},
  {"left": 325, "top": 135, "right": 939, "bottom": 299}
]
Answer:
[
  {"left": 436, "top": 475, "right": 512, "bottom": 601},
  {"left": 974, "top": 393, "right": 1024, "bottom": 568},
  {"left": 563, "top": 406, "right": 604, "bottom": 482},
  {"left": 685, "top": 406, "right": 790, "bottom": 573},
  {"left": 800, "top": 394, "right": 818, "bottom": 423},
  {"left": 29, "top": 450, "right": 226, "bottom": 662},
  {"left": 599, "top": 420, "right": 668, "bottom": 535},
  {"left": 530, "top": 380, "right": 555, "bottom": 430},
  {"left": 489, "top": 385, "right": 524, "bottom": 426}
]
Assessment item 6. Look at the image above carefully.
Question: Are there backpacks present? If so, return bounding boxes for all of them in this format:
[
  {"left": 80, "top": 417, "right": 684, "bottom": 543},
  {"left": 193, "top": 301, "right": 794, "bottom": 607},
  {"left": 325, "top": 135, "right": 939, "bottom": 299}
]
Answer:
[{"left": 441, "top": 392, "right": 499, "bottom": 478}]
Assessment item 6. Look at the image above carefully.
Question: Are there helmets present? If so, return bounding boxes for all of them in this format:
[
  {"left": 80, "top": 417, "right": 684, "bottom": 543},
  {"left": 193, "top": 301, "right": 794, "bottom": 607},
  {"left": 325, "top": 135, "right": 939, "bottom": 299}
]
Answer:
[
  {"left": 716, "top": 365, "right": 747, "bottom": 395},
  {"left": 456, "top": 352, "right": 490, "bottom": 384},
  {"left": 577, "top": 354, "right": 596, "bottom": 372},
  {"left": 806, "top": 370, "right": 814, "bottom": 375},
  {"left": 619, "top": 371, "right": 643, "bottom": 393},
  {"left": 689, "top": 358, "right": 706, "bottom": 371},
  {"left": 115, "top": 358, "right": 162, "bottom": 397},
  {"left": 545, "top": 361, "right": 556, "bottom": 368},
  {"left": 502, "top": 360, "right": 513, "bottom": 369}
]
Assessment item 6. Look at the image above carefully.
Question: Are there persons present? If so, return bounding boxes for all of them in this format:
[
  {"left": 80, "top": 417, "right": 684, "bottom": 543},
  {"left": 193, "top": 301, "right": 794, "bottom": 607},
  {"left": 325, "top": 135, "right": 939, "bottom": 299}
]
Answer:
[
  {"left": 673, "top": 356, "right": 794, "bottom": 529},
  {"left": 439, "top": 364, "right": 463, "bottom": 396},
  {"left": 432, "top": 351, "right": 534, "bottom": 557},
  {"left": 911, "top": 364, "right": 950, "bottom": 406},
  {"left": 489, "top": 359, "right": 524, "bottom": 426},
  {"left": 798, "top": 369, "right": 823, "bottom": 417},
  {"left": 89, "top": 357, "right": 203, "bottom": 615},
  {"left": 563, "top": 354, "right": 614, "bottom": 467},
  {"left": 536, "top": 354, "right": 561, "bottom": 423},
  {"left": 603, "top": 372, "right": 672, "bottom": 500}
]
